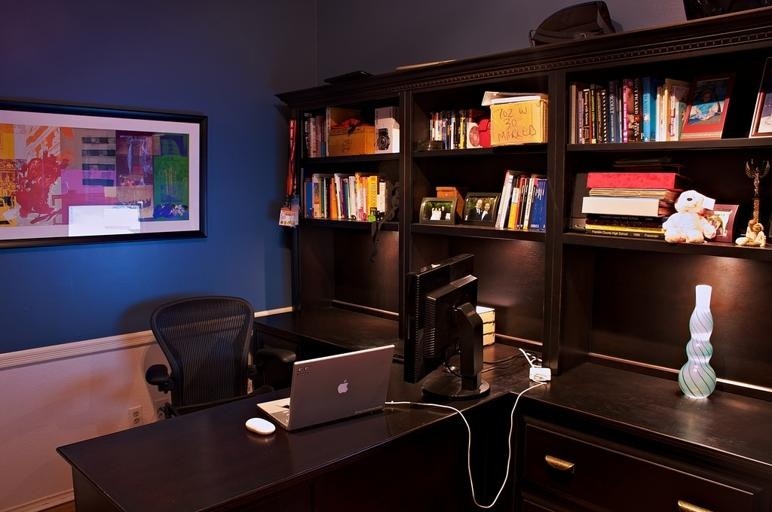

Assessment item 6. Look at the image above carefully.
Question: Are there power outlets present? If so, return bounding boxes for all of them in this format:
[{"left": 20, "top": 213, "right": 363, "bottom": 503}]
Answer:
[{"left": 128, "top": 405, "right": 143, "bottom": 426}]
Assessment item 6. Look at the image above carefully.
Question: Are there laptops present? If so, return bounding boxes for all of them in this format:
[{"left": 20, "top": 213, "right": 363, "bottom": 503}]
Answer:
[{"left": 257, "top": 344, "right": 395, "bottom": 431}]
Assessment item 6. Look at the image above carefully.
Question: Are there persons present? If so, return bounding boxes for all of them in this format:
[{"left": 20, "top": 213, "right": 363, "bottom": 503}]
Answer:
[
  {"left": 467, "top": 199, "right": 492, "bottom": 221},
  {"left": 705, "top": 217, "right": 723, "bottom": 235},
  {"left": 428, "top": 206, "right": 450, "bottom": 220},
  {"left": 688, "top": 87, "right": 719, "bottom": 125}
]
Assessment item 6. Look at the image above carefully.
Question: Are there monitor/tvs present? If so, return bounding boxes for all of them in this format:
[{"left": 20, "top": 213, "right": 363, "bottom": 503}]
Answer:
[{"left": 403, "top": 254, "right": 490, "bottom": 401}]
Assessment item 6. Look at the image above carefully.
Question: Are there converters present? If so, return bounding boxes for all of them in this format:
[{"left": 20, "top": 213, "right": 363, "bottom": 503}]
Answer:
[{"left": 530, "top": 368, "right": 551, "bottom": 382}]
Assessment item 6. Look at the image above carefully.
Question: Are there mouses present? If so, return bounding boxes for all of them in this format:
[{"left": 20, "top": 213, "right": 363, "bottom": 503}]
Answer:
[{"left": 246, "top": 418, "right": 276, "bottom": 435}]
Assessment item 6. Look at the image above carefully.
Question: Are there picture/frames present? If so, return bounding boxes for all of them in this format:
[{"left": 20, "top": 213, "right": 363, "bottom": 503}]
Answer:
[{"left": 0, "top": 98, "right": 208, "bottom": 249}]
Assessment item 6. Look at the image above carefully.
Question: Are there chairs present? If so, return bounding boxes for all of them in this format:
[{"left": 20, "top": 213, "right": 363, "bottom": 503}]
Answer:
[{"left": 145, "top": 296, "right": 298, "bottom": 420}]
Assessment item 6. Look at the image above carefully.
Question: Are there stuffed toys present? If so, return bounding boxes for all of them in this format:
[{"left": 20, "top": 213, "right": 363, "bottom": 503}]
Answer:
[{"left": 661, "top": 190, "right": 716, "bottom": 243}]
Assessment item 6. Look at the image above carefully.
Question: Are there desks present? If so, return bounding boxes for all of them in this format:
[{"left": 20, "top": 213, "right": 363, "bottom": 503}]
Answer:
[{"left": 56, "top": 361, "right": 524, "bottom": 511}]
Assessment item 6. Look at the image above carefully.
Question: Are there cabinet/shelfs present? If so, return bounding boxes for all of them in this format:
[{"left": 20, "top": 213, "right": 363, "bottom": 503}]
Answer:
[{"left": 253, "top": 6, "right": 772, "bottom": 512}]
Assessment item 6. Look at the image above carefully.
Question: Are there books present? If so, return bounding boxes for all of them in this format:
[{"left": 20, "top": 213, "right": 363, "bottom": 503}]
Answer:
[
  {"left": 473, "top": 301, "right": 499, "bottom": 350},
  {"left": 428, "top": 108, "right": 482, "bottom": 149},
  {"left": 303, "top": 106, "right": 400, "bottom": 157},
  {"left": 494, "top": 170, "right": 547, "bottom": 232},
  {"left": 571, "top": 158, "right": 697, "bottom": 238},
  {"left": 300, "top": 168, "right": 399, "bottom": 221},
  {"left": 569, "top": 77, "right": 691, "bottom": 144}
]
